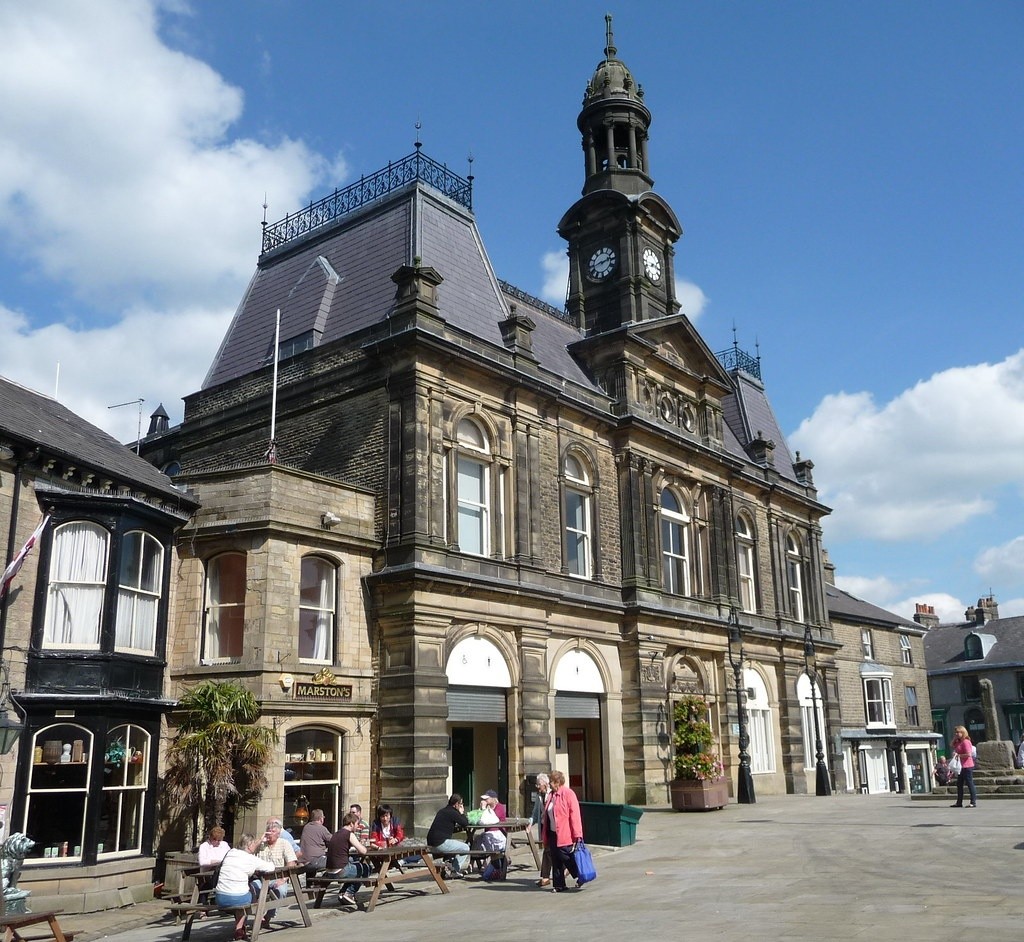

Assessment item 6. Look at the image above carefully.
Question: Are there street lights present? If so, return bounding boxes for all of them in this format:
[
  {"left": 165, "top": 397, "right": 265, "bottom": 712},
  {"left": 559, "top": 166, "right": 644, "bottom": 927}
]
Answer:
[
  {"left": 727, "top": 605, "right": 757, "bottom": 805},
  {"left": 803, "top": 622, "right": 831, "bottom": 796}
]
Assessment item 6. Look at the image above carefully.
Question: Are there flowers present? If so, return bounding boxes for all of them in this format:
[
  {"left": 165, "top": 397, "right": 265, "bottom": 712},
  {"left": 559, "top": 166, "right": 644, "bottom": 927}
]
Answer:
[{"left": 673, "top": 697, "right": 725, "bottom": 781}]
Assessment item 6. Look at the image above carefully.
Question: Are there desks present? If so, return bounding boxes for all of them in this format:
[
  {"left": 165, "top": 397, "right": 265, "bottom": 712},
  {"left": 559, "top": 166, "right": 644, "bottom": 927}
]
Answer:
[
  {"left": 453, "top": 818, "right": 543, "bottom": 880},
  {"left": 174, "top": 861, "right": 220, "bottom": 925},
  {"left": 182, "top": 866, "right": 311, "bottom": 942},
  {"left": 0, "top": 909, "right": 67, "bottom": 942},
  {"left": 314, "top": 846, "right": 450, "bottom": 912}
]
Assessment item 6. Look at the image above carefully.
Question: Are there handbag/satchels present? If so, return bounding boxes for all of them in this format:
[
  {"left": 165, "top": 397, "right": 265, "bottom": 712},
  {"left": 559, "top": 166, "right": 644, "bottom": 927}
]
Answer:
[
  {"left": 210, "top": 849, "right": 231, "bottom": 888},
  {"left": 483, "top": 853, "right": 504, "bottom": 881},
  {"left": 574, "top": 840, "right": 597, "bottom": 883},
  {"left": 948, "top": 753, "right": 962, "bottom": 774}
]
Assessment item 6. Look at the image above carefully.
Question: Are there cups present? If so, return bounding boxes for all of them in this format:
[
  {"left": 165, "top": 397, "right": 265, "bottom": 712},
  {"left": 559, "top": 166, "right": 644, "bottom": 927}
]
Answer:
[
  {"left": 286, "top": 753, "right": 304, "bottom": 763},
  {"left": 306, "top": 748, "right": 333, "bottom": 763},
  {"left": 370, "top": 843, "right": 380, "bottom": 851}
]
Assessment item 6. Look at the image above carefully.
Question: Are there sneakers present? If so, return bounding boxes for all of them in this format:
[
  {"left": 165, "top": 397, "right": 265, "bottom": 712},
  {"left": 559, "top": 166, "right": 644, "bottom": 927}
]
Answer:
[
  {"left": 343, "top": 892, "right": 357, "bottom": 905},
  {"left": 338, "top": 893, "right": 346, "bottom": 904}
]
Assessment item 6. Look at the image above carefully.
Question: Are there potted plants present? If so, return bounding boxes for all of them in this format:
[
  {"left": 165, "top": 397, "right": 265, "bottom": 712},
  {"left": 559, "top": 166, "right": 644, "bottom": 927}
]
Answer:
[{"left": 157, "top": 677, "right": 281, "bottom": 898}]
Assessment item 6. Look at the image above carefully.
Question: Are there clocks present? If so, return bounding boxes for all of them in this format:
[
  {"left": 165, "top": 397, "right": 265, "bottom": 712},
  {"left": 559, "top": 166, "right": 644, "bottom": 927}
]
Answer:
[
  {"left": 642, "top": 247, "right": 661, "bottom": 281},
  {"left": 587, "top": 246, "right": 616, "bottom": 278}
]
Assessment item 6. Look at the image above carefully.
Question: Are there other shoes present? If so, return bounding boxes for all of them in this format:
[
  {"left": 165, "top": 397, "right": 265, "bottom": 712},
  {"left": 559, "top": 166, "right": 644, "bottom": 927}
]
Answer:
[
  {"left": 536, "top": 878, "right": 550, "bottom": 886},
  {"left": 575, "top": 878, "right": 584, "bottom": 888},
  {"left": 965, "top": 803, "right": 976, "bottom": 808},
  {"left": 552, "top": 887, "right": 567, "bottom": 892},
  {"left": 451, "top": 871, "right": 464, "bottom": 879},
  {"left": 951, "top": 804, "right": 962, "bottom": 807}
]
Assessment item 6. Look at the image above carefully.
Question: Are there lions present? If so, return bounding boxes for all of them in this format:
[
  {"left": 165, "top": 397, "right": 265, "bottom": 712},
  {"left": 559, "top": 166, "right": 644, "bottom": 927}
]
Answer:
[{"left": 1, "top": 832, "right": 35, "bottom": 894}]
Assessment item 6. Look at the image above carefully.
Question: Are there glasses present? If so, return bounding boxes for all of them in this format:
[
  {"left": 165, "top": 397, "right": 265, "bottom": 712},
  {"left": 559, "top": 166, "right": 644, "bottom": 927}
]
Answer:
[{"left": 955, "top": 731, "right": 962, "bottom": 733}]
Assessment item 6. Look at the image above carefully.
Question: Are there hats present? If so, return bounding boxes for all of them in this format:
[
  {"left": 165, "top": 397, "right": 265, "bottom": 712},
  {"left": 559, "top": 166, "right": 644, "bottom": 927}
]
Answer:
[{"left": 481, "top": 790, "right": 497, "bottom": 799}]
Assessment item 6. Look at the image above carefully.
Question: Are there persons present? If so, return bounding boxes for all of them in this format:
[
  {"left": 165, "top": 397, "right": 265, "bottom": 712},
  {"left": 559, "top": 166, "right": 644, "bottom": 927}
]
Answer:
[
  {"left": 931, "top": 755, "right": 951, "bottom": 783},
  {"left": 249, "top": 818, "right": 302, "bottom": 928},
  {"left": 349, "top": 804, "right": 369, "bottom": 861},
  {"left": 1015, "top": 733, "right": 1024, "bottom": 769},
  {"left": 371, "top": 804, "right": 403, "bottom": 868},
  {"left": 301, "top": 809, "right": 333, "bottom": 900},
  {"left": 480, "top": 789, "right": 512, "bottom": 869},
  {"left": 427, "top": 794, "right": 470, "bottom": 879},
  {"left": 198, "top": 827, "right": 231, "bottom": 920},
  {"left": 215, "top": 833, "right": 275, "bottom": 939},
  {"left": 326, "top": 814, "right": 371, "bottom": 911},
  {"left": 526, "top": 771, "right": 583, "bottom": 893},
  {"left": 950, "top": 725, "right": 976, "bottom": 807}
]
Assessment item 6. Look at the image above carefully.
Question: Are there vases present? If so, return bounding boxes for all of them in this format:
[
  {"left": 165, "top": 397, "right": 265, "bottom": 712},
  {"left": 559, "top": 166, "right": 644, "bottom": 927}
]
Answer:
[{"left": 670, "top": 777, "right": 729, "bottom": 812}]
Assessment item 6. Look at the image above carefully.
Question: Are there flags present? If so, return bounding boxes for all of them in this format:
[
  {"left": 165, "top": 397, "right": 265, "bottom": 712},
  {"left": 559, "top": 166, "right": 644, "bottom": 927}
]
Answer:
[{"left": 0, "top": 523, "right": 35, "bottom": 603}]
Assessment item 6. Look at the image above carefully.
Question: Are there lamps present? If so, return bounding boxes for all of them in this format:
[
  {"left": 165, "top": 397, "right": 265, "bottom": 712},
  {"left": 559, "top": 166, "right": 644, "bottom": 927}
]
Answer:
[{"left": 323, "top": 512, "right": 342, "bottom": 526}]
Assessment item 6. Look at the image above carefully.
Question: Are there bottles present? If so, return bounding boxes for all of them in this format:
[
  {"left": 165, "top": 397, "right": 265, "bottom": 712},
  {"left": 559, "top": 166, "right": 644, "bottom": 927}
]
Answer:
[{"left": 33, "top": 746, "right": 43, "bottom": 763}]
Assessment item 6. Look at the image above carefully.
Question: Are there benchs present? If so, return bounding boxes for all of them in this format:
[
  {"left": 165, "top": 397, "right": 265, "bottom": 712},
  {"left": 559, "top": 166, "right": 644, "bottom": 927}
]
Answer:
[
  {"left": 162, "top": 840, "right": 545, "bottom": 915},
  {"left": 0, "top": 930, "right": 85, "bottom": 942}
]
